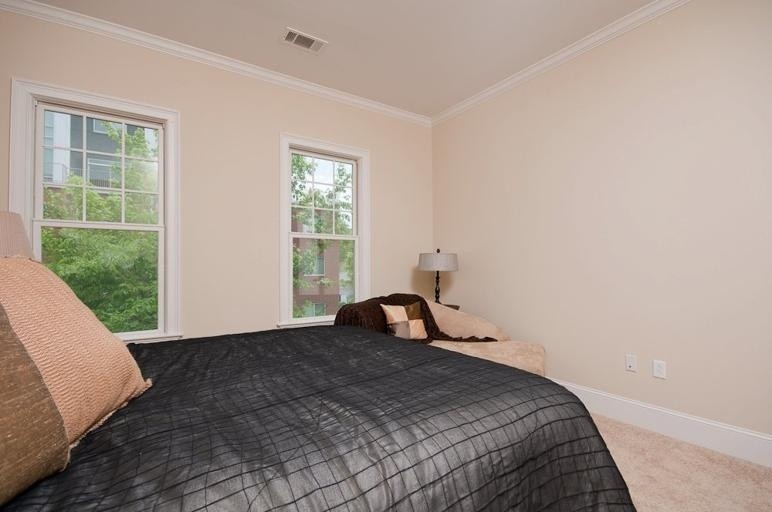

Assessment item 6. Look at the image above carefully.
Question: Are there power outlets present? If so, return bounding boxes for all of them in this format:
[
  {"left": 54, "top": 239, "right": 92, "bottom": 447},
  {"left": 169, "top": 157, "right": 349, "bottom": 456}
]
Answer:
[{"left": 626, "top": 355, "right": 637, "bottom": 372}]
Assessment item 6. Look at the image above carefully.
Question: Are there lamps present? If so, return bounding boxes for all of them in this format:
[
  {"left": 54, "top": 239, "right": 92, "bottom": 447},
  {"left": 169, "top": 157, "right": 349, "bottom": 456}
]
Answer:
[{"left": 418, "top": 248, "right": 460, "bottom": 304}]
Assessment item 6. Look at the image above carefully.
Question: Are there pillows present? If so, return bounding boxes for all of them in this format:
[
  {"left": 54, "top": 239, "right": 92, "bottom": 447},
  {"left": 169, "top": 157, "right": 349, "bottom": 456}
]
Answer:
[
  {"left": 378, "top": 301, "right": 430, "bottom": 342},
  {"left": 2, "top": 249, "right": 152, "bottom": 512}
]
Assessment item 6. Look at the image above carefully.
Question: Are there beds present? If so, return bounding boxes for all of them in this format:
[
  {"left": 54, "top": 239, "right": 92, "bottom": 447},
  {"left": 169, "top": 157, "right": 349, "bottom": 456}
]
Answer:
[{"left": 2, "top": 210, "right": 636, "bottom": 511}]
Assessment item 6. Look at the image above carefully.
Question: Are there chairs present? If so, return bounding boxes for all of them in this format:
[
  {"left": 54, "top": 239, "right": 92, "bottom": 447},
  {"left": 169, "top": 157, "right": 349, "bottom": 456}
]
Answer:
[{"left": 334, "top": 291, "right": 549, "bottom": 378}]
